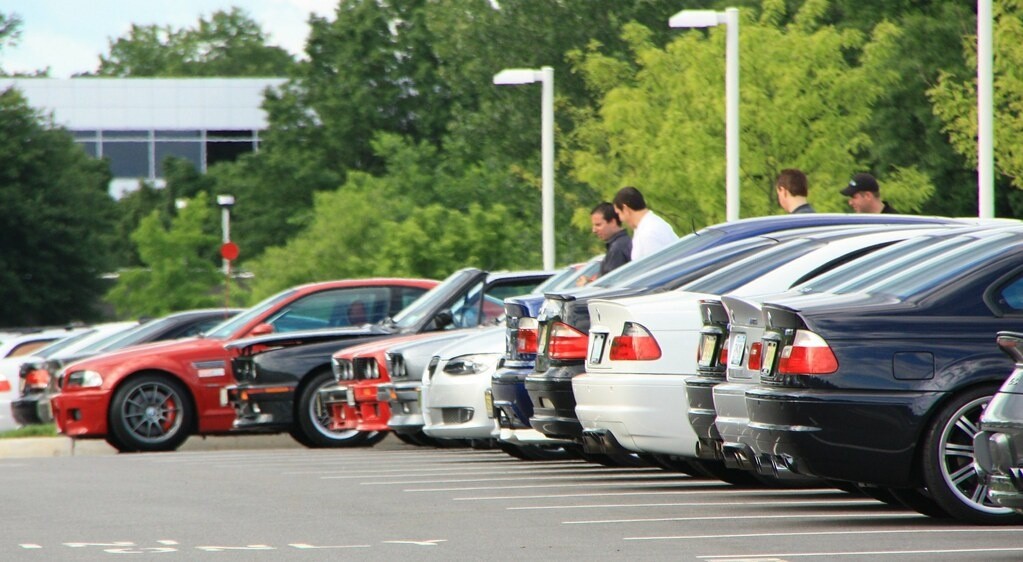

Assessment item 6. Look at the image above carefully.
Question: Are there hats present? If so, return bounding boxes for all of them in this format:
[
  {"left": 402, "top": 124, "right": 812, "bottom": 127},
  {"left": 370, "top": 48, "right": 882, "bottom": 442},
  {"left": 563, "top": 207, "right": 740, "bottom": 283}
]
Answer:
[{"left": 840, "top": 174, "right": 879, "bottom": 196}]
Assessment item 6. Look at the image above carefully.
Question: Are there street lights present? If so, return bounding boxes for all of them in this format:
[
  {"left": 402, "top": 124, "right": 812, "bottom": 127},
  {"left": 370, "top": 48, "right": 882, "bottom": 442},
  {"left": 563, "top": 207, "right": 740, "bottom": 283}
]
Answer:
[
  {"left": 664, "top": 6, "right": 742, "bottom": 223},
  {"left": 216, "top": 193, "right": 237, "bottom": 275},
  {"left": 490, "top": 63, "right": 560, "bottom": 273}
]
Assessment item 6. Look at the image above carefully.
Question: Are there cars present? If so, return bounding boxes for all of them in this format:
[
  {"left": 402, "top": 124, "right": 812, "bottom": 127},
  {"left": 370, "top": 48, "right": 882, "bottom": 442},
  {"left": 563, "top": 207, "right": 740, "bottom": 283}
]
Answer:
[
  {"left": 0, "top": 316, "right": 223, "bottom": 436},
  {"left": 49, "top": 275, "right": 507, "bottom": 455},
  {"left": 219, "top": 209, "right": 1023, "bottom": 528},
  {"left": 970, "top": 329, "right": 1023, "bottom": 518},
  {"left": 7, "top": 307, "right": 343, "bottom": 435}
]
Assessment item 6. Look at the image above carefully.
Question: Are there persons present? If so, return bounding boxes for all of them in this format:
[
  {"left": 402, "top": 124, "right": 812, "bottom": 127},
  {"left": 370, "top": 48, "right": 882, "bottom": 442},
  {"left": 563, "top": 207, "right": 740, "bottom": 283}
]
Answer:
[
  {"left": 839, "top": 173, "right": 901, "bottom": 215},
  {"left": 775, "top": 168, "right": 813, "bottom": 216},
  {"left": 348, "top": 300, "right": 366, "bottom": 325},
  {"left": 613, "top": 187, "right": 682, "bottom": 263},
  {"left": 577, "top": 201, "right": 632, "bottom": 284}
]
